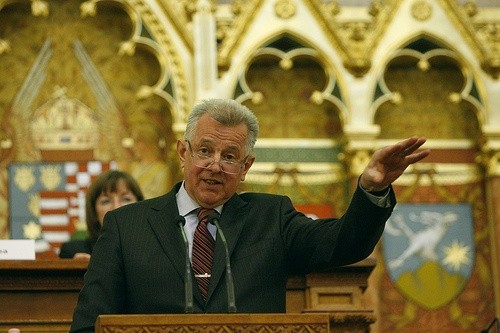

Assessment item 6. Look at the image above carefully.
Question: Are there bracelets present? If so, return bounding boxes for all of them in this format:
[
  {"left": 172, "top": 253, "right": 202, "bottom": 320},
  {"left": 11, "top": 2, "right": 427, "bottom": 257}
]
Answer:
[{"left": 360, "top": 183, "right": 390, "bottom": 195}]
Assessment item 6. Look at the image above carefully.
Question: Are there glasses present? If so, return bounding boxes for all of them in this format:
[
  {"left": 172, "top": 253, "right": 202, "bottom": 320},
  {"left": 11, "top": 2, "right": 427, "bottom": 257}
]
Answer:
[{"left": 184, "top": 139, "right": 249, "bottom": 175}]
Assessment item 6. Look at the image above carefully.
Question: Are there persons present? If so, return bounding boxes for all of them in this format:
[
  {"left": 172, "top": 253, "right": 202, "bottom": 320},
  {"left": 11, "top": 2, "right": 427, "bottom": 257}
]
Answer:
[
  {"left": 70, "top": 98, "right": 431, "bottom": 333},
  {"left": 59, "top": 169, "right": 147, "bottom": 259}
]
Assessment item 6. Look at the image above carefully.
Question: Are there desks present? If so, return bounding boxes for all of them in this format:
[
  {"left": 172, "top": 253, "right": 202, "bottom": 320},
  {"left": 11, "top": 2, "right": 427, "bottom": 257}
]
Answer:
[{"left": 0, "top": 257, "right": 378, "bottom": 333}]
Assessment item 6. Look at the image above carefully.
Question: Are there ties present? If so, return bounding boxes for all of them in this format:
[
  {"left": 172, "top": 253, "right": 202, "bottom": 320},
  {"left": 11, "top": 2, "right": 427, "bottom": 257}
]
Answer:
[{"left": 191, "top": 207, "right": 216, "bottom": 302}]
